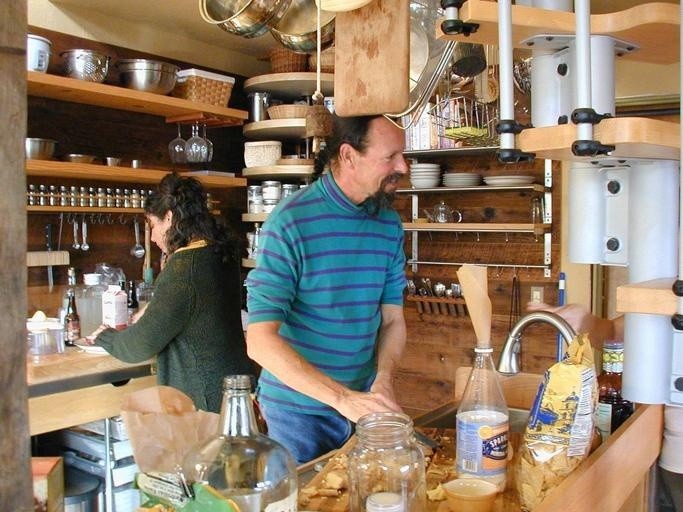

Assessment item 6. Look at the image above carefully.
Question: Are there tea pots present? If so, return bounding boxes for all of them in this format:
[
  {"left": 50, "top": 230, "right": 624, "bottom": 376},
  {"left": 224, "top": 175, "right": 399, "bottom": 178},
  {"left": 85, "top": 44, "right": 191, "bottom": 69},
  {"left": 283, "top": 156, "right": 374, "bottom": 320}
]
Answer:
[{"left": 422, "top": 201, "right": 463, "bottom": 222}]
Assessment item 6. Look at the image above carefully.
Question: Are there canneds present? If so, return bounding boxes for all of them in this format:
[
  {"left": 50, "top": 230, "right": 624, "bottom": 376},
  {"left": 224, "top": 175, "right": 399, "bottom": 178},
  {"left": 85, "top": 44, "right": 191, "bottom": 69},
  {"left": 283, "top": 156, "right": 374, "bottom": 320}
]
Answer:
[{"left": 246, "top": 180, "right": 309, "bottom": 213}]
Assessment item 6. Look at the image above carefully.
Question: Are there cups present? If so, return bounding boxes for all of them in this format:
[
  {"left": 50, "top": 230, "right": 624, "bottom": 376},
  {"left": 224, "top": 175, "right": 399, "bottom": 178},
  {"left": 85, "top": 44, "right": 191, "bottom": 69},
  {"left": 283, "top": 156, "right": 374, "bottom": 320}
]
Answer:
[
  {"left": 413, "top": 217, "right": 429, "bottom": 223},
  {"left": 130, "top": 160, "right": 142, "bottom": 168},
  {"left": 446, "top": 478, "right": 496, "bottom": 511},
  {"left": 103, "top": 157, "right": 119, "bottom": 166}
]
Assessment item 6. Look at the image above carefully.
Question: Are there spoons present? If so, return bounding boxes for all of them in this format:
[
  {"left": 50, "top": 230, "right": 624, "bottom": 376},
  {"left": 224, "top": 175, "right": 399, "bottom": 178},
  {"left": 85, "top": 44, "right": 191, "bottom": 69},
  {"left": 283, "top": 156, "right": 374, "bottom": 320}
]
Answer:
[
  {"left": 72, "top": 221, "right": 80, "bottom": 250},
  {"left": 134, "top": 222, "right": 145, "bottom": 259},
  {"left": 81, "top": 219, "right": 89, "bottom": 250}
]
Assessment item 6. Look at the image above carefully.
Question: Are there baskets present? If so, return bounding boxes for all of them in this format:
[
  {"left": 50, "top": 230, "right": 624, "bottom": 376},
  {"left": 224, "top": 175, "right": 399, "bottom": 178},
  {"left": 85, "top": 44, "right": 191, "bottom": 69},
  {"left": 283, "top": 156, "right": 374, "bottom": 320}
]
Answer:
[{"left": 169, "top": 68, "right": 237, "bottom": 111}]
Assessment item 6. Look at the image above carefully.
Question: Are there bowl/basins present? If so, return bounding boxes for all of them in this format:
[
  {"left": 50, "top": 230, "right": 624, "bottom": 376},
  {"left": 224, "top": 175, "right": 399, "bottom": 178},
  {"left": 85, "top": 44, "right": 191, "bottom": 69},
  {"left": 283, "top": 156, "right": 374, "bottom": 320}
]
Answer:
[
  {"left": 115, "top": 59, "right": 179, "bottom": 93},
  {"left": 24, "top": 138, "right": 58, "bottom": 159},
  {"left": 197, "top": 0, "right": 371, "bottom": 55},
  {"left": 27, "top": 316, "right": 63, "bottom": 356}
]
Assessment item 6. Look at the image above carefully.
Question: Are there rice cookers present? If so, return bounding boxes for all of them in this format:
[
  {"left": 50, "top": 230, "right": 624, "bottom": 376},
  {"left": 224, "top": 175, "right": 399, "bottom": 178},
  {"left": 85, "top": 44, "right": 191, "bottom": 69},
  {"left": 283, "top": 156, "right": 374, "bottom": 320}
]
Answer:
[{"left": 27, "top": 32, "right": 53, "bottom": 73}]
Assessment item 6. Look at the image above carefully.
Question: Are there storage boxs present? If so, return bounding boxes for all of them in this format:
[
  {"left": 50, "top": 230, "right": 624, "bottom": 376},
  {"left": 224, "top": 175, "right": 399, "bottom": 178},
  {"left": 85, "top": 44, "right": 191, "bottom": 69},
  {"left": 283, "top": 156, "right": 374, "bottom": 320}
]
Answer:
[{"left": 28, "top": 450, "right": 68, "bottom": 512}]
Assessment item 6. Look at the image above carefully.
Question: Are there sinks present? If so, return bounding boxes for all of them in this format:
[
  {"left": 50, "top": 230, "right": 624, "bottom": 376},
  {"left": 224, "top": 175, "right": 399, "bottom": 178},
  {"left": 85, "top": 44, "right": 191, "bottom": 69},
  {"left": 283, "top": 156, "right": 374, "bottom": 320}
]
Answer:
[{"left": 411, "top": 399, "right": 534, "bottom": 434}]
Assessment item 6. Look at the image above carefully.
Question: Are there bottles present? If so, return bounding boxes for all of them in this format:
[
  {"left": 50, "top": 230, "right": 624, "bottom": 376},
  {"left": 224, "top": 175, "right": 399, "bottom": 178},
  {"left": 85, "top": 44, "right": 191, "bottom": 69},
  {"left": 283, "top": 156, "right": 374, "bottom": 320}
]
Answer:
[
  {"left": 247, "top": 91, "right": 334, "bottom": 122},
  {"left": 62, "top": 260, "right": 137, "bottom": 348},
  {"left": 457, "top": 342, "right": 509, "bottom": 493},
  {"left": 347, "top": 409, "right": 426, "bottom": 512},
  {"left": 179, "top": 374, "right": 297, "bottom": 512},
  {"left": 249, "top": 180, "right": 312, "bottom": 216},
  {"left": 28, "top": 185, "right": 151, "bottom": 209},
  {"left": 599, "top": 338, "right": 632, "bottom": 438}
]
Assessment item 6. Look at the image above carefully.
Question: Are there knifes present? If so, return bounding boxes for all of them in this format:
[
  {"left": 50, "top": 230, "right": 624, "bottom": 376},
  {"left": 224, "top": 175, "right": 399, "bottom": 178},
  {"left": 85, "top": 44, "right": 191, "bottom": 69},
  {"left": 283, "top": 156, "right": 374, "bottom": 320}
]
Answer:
[{"left": 44, "top": 223, "right": 57, "bottom": 294}]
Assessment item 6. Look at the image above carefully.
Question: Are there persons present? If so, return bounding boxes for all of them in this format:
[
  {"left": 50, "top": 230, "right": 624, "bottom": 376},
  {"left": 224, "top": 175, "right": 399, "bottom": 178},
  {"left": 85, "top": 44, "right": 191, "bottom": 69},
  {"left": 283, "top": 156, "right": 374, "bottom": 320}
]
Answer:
[
  {"left": 244, "top": 115, "right": 409, "bottom": 484},
  {"left": 526, "top": 300, "right": 682, "bottom": 511},
  {"left": 89, "top": 172, "right": 258, "bottom": 490}
]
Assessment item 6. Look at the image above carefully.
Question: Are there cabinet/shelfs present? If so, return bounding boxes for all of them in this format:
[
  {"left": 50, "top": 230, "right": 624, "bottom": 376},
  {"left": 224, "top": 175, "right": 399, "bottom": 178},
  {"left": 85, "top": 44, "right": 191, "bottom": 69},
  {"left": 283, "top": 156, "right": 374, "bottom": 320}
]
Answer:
[
  {"left": 240, "top": 70, "right": 344, "bottom": 282},
  {"left": 25, "top": 68, "right": 250, "bottom": 221},
  {"left": 284, "top": 360, "right": 666, "bottom": 511},
  {"left": 365, "top": 143, "right": 558, "bottom": 284},
  {"left": 26, "top": 330, "right": 164, "bottom": 512}
]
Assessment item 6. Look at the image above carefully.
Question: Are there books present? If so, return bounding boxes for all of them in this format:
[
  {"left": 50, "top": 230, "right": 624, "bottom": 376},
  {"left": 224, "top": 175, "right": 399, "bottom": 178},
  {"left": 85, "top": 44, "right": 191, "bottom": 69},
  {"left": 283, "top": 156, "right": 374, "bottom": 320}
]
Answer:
[{"left": 401, "top": 94, "right": 461, "bottom": 153}]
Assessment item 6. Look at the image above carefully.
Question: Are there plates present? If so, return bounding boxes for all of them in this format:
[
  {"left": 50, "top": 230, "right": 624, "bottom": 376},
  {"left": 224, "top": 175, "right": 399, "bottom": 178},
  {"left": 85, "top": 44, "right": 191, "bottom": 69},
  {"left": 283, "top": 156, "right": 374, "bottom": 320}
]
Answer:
[
  {"left": 67, "top": 154, "right": 96, "bottom": 163},
  {"left": 408, "top": 160, "right": 535, "bottom": 188}
]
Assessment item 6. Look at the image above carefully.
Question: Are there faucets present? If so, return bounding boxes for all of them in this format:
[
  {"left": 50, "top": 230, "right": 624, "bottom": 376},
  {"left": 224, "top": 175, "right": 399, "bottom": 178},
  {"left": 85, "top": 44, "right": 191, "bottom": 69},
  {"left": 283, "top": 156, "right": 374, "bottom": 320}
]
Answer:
[{"left": 497, "top": 310, "right": 579, "bottom": 375}]
